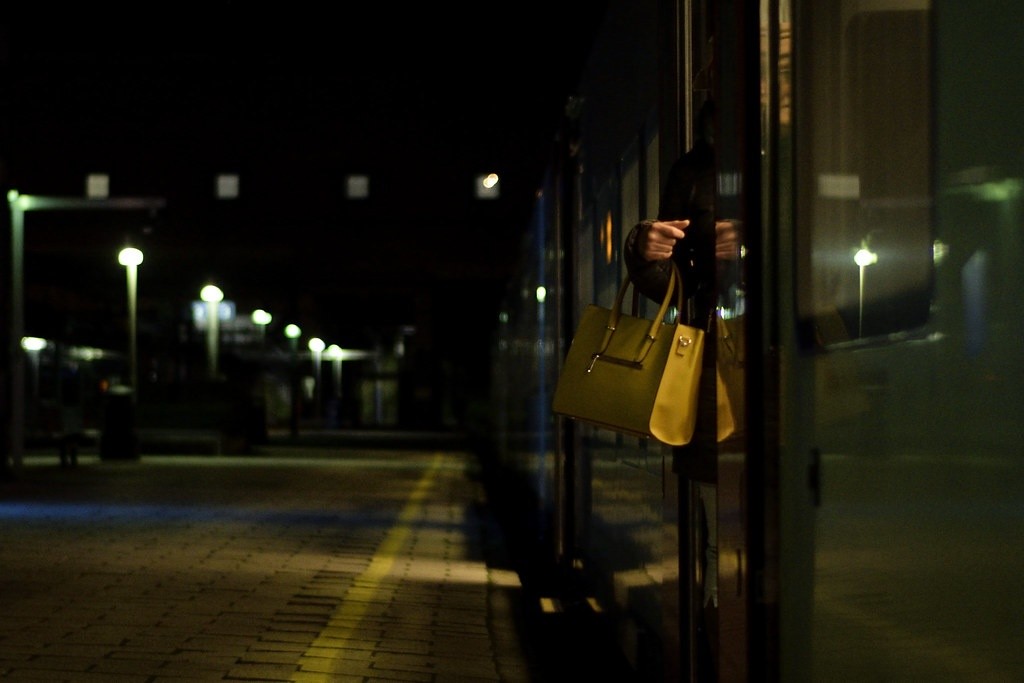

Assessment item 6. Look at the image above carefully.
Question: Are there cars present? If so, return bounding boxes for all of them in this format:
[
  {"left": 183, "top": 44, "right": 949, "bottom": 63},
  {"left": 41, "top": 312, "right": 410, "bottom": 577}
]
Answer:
[{"left": 96, "top": 352, "right": 261, "bottom": 457}]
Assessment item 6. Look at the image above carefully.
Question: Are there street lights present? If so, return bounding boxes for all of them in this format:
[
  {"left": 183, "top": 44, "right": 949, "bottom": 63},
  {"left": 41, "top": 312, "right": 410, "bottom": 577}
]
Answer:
[
  {"left": 119, "top": 248, "right": 143, "bottom": 380},
  {"left": 308, "top": 338, "right": 325, "bottom": 387},
  {"left": 285, "top": 325, "right": 302, "bottom": 371},
  {"left": 252, "top": 309, "right": 266, "bottom": 354},
  {"left": 328, "top": 344, "right": 344, "bottom": 391},
  {"left": 200, "top": 286, "right": 225, "bottom": 371}
]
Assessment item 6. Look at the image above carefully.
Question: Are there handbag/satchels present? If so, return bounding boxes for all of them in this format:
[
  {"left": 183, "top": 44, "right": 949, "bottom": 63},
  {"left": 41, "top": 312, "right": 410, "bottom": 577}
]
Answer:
[
  {"left": 713, "top": 367, "right": 737, "bottom": 441},
  {"left": 549, "top": 261, "right": 705, "bottom": 448}
]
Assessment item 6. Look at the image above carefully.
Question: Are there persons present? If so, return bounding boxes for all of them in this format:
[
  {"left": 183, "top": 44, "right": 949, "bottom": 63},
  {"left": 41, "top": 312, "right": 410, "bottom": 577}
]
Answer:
[{"left": 624, "top": 36, "right": 716, "bottom": 609}]
